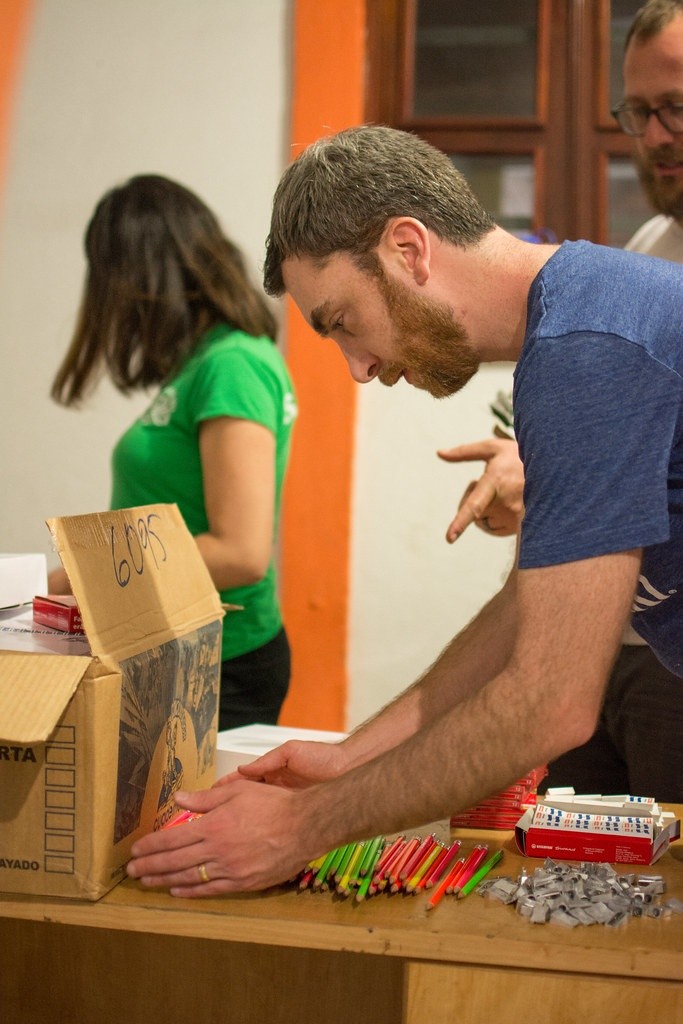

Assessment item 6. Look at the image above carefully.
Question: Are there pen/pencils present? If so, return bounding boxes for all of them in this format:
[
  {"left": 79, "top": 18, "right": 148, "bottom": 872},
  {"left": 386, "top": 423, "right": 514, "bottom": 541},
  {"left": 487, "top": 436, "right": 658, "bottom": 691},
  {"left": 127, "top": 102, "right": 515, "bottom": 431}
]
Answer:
[
  {"left": 160, "top": 810, "right": 204, "bottom": 829},
  {"left": 445, "top": 843, "right": 482, "bottom": 896},
  {"left": 295, "top": 832, "right": 462, "bottom": 903},
  {"left": 453, "top": 845, "right": 489, "bottom": 894},
  {"left": 456, "top": 848, "right": 504, "bottom": 900},
  {"left": 424, "top": 857, "right": 465, "bottom": 911}
]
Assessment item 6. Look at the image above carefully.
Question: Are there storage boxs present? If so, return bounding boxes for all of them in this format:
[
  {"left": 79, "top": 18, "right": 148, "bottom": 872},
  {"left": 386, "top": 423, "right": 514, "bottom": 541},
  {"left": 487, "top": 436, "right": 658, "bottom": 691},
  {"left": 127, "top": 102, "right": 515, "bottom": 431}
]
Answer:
[
  {"left": 217, "top": 723, "right": 348, "bottom": 777},
  {"left": 1, "top": 503, "right": 225, "bottom": 902},
  {"left": 514, "top": 786, "right": 680, "bottom": 865}
]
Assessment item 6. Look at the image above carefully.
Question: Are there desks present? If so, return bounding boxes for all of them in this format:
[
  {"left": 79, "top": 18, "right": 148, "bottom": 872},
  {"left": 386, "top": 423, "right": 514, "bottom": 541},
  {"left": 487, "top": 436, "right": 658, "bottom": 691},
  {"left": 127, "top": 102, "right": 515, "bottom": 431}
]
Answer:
[{"left": 0, "top": 794, "right": 683, "bottom": 1024}]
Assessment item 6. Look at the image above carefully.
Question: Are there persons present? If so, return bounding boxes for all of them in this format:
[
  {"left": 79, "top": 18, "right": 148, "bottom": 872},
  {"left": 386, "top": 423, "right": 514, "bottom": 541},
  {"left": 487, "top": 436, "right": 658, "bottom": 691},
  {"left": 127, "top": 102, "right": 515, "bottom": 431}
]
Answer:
[
  {"left": 127, "top": 128, "right": 683, "bottom": 901},
  {"left": 438, "top": 1, "right": 683, "bottom": 805},
  {"left": 48, "top": 174, "right": 296, "bottom": 731}
]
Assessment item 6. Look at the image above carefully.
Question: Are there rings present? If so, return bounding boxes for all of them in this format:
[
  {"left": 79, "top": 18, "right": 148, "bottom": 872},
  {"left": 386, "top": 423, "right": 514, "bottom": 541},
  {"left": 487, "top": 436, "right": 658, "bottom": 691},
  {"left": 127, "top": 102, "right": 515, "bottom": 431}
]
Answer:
[{"left": 198, "top": 864, "right": 210, "bottom": 883}]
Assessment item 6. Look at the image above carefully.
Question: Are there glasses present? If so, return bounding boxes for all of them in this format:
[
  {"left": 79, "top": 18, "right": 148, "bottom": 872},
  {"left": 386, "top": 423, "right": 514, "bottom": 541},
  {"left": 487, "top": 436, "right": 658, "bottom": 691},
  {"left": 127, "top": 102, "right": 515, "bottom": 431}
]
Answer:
[{"left": 609, "top": 103, "right": 683, "bottom": 136}]
